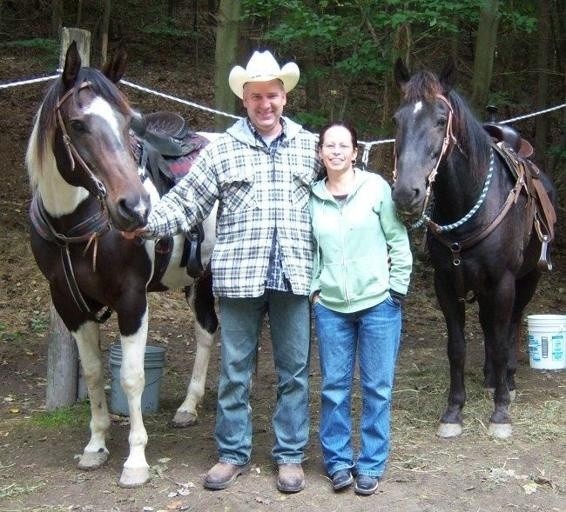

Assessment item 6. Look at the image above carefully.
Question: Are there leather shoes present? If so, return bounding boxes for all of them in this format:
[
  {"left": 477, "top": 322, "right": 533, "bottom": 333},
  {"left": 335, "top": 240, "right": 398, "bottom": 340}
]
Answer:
[
  {"left": 331, "top": 469, "right": 354, "bottom": 491},
  {"left": 355, "top": 475, "right": 379, "bottom": 495},
  {"left": 203, "top": 461, "right": 251, "bottom": 489},
  {"left": 275, "top": 463, "right": 305, "bottom": 494}
]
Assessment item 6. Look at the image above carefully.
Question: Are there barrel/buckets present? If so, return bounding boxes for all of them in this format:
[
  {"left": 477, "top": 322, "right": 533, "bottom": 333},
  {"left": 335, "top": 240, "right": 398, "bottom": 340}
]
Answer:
[
  {"left": 527, "top": 314, "right": 566, "bottom": 370},
  {"left": 108, "top": 345, "right": 168, "bottom": 417}
]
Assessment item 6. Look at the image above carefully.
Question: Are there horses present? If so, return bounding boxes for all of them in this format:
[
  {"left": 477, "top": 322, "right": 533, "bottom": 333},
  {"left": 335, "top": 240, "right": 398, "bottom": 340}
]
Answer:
[
  {"left": 25, "top": 38, "right": 224, "bottom": 488},
  {"left": 391, "top": 57, "right": 556, "bottom": 440}
]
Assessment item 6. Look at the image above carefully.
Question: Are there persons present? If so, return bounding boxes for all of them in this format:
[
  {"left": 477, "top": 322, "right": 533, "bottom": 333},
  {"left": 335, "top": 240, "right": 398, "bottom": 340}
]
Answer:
[
  {"left": 121, "top": 51, "right": 328, "bottom": 496},
  {"left": 305, "top": 120, "right": 413, "bottom": 497}
]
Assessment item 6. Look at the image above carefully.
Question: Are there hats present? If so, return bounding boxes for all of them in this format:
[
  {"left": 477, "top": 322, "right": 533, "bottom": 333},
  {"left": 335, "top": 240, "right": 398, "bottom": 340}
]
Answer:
[{"left": 228, "top": 50, "right": 300, "bottom": 100}]
